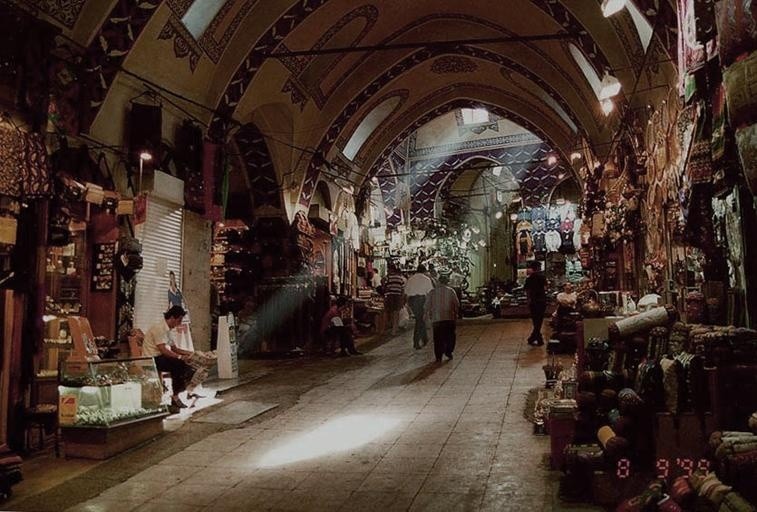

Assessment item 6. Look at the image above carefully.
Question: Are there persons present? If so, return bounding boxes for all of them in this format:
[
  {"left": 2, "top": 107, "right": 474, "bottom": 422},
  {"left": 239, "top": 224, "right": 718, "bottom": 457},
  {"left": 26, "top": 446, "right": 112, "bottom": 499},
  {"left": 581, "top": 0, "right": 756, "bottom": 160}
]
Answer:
[
  {"left": 142, "top": 305, "right": 194, "bottom": 408},
  {"left": 369, "top": 264, "right": 460, "bottom": 363},
  {"left": 319, "top": 298, "right": 363, "bottom": 357},
  {"left": 166, "top": 270, "right": 194, "bottom": 352},
  {"left": 525, "top": 262, "right": 548, "bottom": 345},
  {"left": 552, "top": 282, "right": 577, "bottom": 317}
]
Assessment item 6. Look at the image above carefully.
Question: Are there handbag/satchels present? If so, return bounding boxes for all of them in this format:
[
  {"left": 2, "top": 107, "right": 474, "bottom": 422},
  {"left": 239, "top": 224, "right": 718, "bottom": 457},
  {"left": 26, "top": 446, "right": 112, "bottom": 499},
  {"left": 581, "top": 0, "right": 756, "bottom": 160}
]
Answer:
[
  {"left": 117, "top": 236, "right": 143, "bottom": 283},
  {"left": 64, "top": 179, "right": 134, "bottom": 215}
]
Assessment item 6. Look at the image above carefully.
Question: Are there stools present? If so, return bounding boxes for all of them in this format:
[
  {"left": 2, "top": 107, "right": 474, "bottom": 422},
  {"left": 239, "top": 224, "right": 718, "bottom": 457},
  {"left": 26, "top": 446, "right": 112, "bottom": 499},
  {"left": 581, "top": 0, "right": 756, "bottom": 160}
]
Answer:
[
  {"left": 321, "top": 336, "right": 340, "bottom": 355},
  {"left": 25, "top": 403, "right": 59, "bottom": 451}
]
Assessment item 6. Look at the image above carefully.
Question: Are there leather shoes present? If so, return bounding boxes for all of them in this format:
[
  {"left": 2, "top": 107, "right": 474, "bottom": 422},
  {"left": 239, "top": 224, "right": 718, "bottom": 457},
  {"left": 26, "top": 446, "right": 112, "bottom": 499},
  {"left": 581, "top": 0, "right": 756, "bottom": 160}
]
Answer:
[
  {"left": 172, "top": 397, "right": 188, "bottom": 408},
  {"left": 187, "top": 393, "right": 207, "bottom": 399}
]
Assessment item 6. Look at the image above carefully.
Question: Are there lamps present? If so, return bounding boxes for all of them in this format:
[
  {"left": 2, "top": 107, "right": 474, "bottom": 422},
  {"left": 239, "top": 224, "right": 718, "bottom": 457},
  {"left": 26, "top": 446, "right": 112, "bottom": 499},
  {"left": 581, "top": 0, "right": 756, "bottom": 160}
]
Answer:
[
  {"left": 599, "top": 70, "right": 622, "bottom": 100},
  {"left": 600, "top": 0, "right": 627, "bottom": 17}
]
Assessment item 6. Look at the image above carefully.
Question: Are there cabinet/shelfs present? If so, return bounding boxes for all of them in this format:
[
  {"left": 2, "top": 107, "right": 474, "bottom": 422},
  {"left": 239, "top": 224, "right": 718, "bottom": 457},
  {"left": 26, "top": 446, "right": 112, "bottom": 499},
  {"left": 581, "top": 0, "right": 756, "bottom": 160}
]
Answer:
[{"left": 58, "top": 357, "right": 171, "bottom": 462}]
